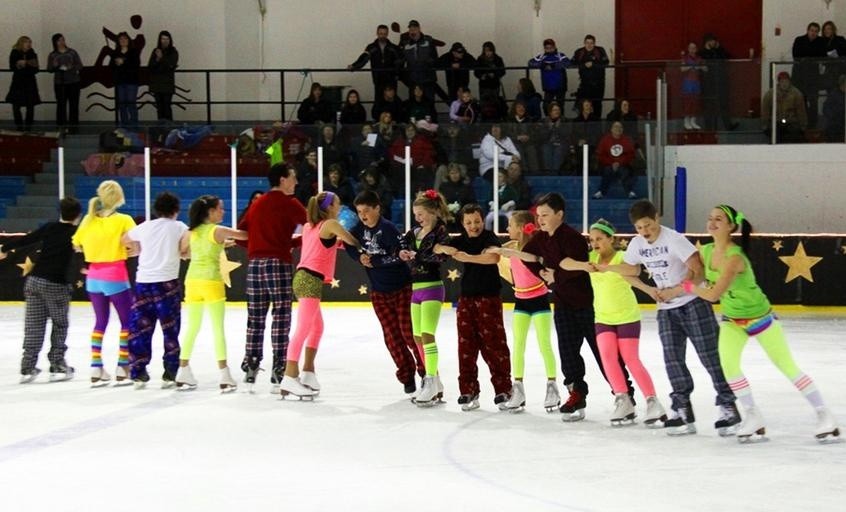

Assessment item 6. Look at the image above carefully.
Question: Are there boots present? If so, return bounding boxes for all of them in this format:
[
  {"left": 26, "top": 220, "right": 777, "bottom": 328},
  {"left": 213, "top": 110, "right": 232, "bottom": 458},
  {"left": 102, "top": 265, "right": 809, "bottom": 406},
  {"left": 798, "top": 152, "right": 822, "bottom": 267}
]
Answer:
[
  {"left": 543, "top": 380, "right": 560, "bottom": 408},
  {"left": 737, "top": 414, "right": 765, "bottom": 438},
  {"left": 218, "top": 365, "right": 320, "bottom": 396},
  {"left": 644, "top": 396, "right": 667, "bottom": 423},
  {"left": 814, "top": 408, "right": 840, "bottom": 438},
  {"left": 610, "top": 393, "right": 635, "bottom": 421},
  {"left": 504, "top": 381, "right": 525, "bottom": 409}
]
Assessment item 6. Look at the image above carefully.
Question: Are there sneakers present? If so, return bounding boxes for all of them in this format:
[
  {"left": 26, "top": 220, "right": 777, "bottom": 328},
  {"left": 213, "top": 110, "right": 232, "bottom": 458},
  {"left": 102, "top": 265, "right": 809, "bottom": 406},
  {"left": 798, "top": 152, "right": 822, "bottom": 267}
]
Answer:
[
  {"left": 458, "top": 392, "right": 479, "bottom": 403},
  {"left": 560, "top": 391, "right": 586, "bottom": 412},
  {"left": 494, "top": 392, "right": 508, "bottom": 404},
  {"left": 20, "top": 365, "right": 198, "bottom": 386},
  {"left": 665, "top": 403, "right": 694, "bottom": 426},
  {"left": 404, "top": 376, "right": 439, "bottom": 403},
  {"left": 715, "top": 401, "right": 741, "bottom": 427}
]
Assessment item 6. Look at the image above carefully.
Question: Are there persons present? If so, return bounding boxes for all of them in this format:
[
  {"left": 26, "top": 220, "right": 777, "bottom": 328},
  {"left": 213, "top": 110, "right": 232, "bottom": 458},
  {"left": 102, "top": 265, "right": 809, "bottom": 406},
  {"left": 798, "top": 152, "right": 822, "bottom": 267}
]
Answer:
[
  {"left": 1, "top": 195, "right": 82, "bottom": 375},
  {"left": 46, "top": 33, "right": 85, "bottom": 123},
  {"left": 72, "top": 180, "right": 141, "bottom": 382},
  {"left": 238, "top": 18, "right": 846, "bottom": 436},
  {"left": 173, "top": 194, "right": 249, "bottom": 389},
  {"left": 121, "top": 191, "right": 190, "bottom": 382},
  {"left": 4, "top": 35, "right": 42, "bottom": 122},
  {"left": 147, "top": 30, "right": 180, "bottom": 120},
  {"left": 108, "top": 31, "right": 141, "bottom": 123}
]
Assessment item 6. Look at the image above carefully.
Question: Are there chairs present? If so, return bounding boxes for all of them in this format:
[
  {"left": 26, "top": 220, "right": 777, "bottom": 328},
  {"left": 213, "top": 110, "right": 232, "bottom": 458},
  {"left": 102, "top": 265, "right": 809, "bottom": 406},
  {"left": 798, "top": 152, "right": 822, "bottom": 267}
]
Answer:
[
  {"left": 390, "top": 124, "right": 651, "bottom": 235},
  {"left": 76, "top": 131, "right": 275, "bottom": 231}
]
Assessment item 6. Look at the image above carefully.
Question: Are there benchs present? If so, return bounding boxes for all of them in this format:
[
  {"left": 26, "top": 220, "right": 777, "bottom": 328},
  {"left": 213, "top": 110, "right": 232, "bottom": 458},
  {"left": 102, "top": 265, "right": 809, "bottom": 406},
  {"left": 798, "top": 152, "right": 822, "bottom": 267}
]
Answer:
[{"left": 0, "top": 126, "right": 53, "bottom": 227}]
